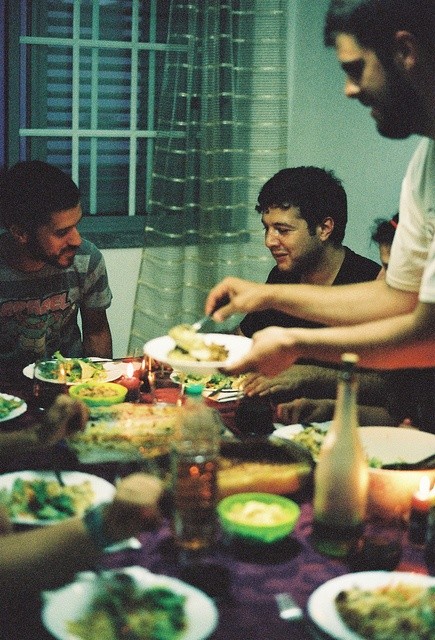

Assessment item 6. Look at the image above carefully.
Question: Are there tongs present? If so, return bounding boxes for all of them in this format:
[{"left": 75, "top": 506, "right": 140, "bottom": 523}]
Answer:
[{"left": 382, "top": 453, "right": 434, "bottom": 470}]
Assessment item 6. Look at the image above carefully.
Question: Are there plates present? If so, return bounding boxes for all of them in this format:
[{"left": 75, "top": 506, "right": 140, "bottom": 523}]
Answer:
[
  {"left": 41, "top": 566, "right": 218, "bottom": 640},
  {"left": 0, "top": 392, "right": 28, "bottom": 423},
  {"left": 22, "top": 354, "right": 125, "bottom": 386},
  {"left": 294, "top": 421, "right": 332, "bottom": 462},
  {"left": 65, "top": 400, "right": 176, "bottom": 467},
  {"left": 0, "top": 470, "right": 117, "bottom": 528},
  {"left": 273, "top": 422, "right": 305, "bottom": 442},
  {"left": 306, "top": 568, "right": 435, "bottom": 639}
]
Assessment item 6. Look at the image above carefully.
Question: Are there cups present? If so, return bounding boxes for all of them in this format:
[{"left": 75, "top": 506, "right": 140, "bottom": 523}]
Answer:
[{"left": 345, "top": 504, "right": 410, "bottom": 571}]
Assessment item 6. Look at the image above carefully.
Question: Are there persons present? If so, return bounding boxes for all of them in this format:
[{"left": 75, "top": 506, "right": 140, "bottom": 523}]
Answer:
[
  {"left": 363, "top": 209, "right": 405, "bottom": 281},
  {"left": 0, "top": 157, "right": 114, "bottom": 370},
  {"left": 203, "top": 1, "right": 431, "bottom": 380},
  {"left": 230, "top": 164, "right": 382, "bottom": 339},
  {"left": 0, "top": 471, "right": 168, "bottom": 592}
]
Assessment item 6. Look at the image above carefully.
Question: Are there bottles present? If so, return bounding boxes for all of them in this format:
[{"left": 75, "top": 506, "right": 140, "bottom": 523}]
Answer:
[
  {"left": 423, "top": 493, "right": 435, "bottom": 576},
  {"left": 409, "top": 473, "right": 433, "bottom": 543},
  {"left": 310, "top": 351, "right": 371, "bottom": 557},
  {"left": 169, "top": 383, "right": 221, "bottom": 550}
]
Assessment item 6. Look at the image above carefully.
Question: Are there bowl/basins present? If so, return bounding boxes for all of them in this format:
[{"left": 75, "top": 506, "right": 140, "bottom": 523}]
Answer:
[
  {"left": 217, "top": 491, "right": 302, "bottom": 549},
  {"left": 142, "top": 332, "right": 253, "bottom": 378},
  {"left": 356, "top": 423, "right": 435, "bottom": 520},
  {"left": 68, "top": 380, "right": 129, "bottom": 407}
]
